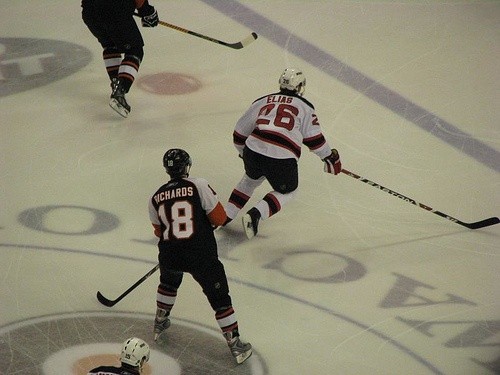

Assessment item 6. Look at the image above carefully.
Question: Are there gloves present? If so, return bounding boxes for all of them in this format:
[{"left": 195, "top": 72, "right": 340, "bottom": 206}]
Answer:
[
  {"left": 139, "top": 0, "right": 159, "bottom": 27},
  {"left": 321, "top": 149, "right": 341, "bottom": 175}
]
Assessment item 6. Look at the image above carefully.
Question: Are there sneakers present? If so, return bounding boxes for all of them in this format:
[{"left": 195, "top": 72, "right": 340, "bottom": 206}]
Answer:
[
  {"left": 242, "top": 207, "right": 261, "bottom": 240},
  {"left": 154, "top": 308, "right": 170, "bottom": 340},
  {"left": 111, "top": 78, "right": 118, "bottom": 92},
  {"left": 110, "top": 79, "right": 130, "bottom": 118},
  {"left": 223, "top": 332, "right": 252, "bottom": 364}
]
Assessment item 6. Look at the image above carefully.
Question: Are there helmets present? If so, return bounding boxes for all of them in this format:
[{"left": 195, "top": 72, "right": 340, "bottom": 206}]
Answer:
[
  {"left": 163, "top": 149, "right": 192, "bottom": 178},
  {"left": 279, "top": 69, "right": 306, "bottom": 96},
  {"left": 120, "top": 337, "right": 150, "bottom": 366}
]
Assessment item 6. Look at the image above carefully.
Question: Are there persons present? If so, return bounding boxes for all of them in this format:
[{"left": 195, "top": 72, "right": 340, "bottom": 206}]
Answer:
[
  {"left": 148, "top": 148, "right": 253, "bottom": 365},
  {"left": 80, "top": 0, "right": 160, "bottom": 117},
  {"left": 87, "top": 337, "right": 150, "bottom": 375},
  {"left": 218, "top": 69, "right": 342, "bottom": 240}
]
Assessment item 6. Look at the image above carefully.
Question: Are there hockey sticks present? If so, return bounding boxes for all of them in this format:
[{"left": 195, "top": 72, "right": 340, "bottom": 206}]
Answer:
[
  {"left": 131, "top": 13, "right": 259, "bottom": 49},
  {"left": 338, "top": 168, "right": 499, "bottom": 231},
  {"left": 96, "top": 222, "right": 215, "bottom": 308}
]
